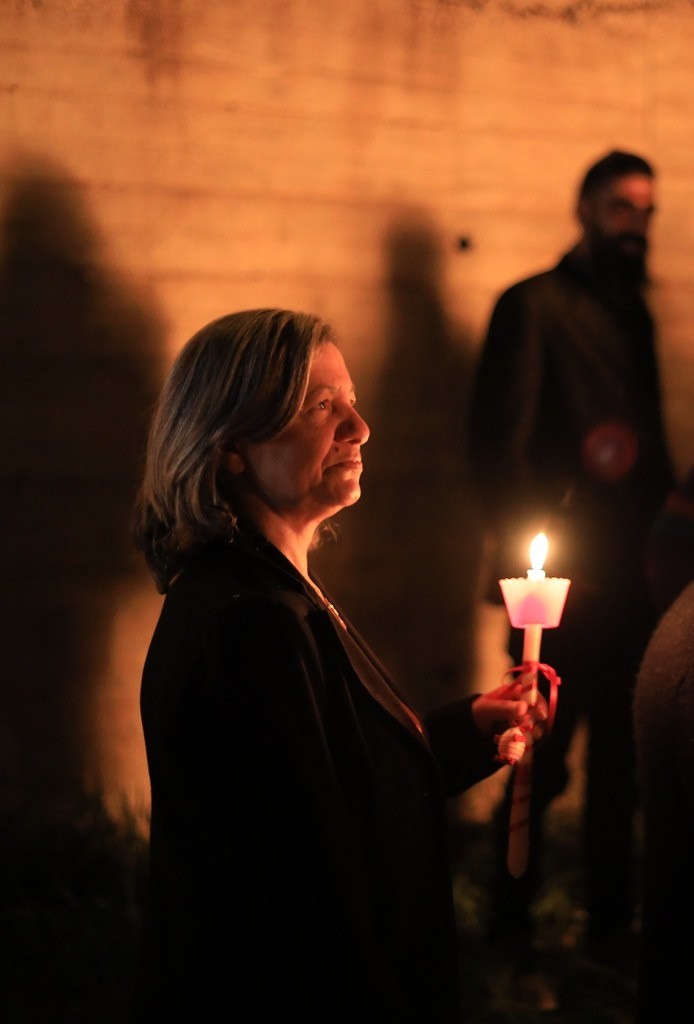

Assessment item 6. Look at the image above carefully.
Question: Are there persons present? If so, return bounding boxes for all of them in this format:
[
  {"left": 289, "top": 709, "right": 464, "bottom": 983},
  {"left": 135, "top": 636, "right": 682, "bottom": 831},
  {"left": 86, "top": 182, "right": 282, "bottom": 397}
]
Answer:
[
  {"left": 470, "top": 151, "right": 694, "bottom": 1023},
  {"left": 130, "top": 307, "right": 550, "bottom": 1024}
]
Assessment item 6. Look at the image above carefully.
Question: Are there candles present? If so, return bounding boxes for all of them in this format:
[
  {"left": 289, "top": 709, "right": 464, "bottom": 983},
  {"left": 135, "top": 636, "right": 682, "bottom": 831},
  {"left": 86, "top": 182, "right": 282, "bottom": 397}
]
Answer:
[{"left": 496, "top": 530, "right": 572, "bottom": 878}]
